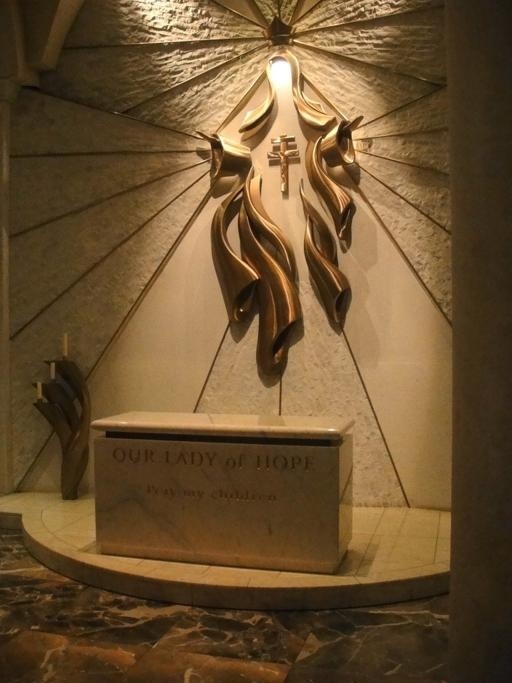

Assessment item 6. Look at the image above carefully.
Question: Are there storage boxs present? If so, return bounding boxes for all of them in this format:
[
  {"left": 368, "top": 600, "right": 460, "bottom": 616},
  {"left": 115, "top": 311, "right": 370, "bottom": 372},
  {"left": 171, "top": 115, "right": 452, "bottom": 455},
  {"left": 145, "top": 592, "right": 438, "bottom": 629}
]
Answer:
[{"left": 90, "top": 411, "right": 355, "bottom": 575}]
